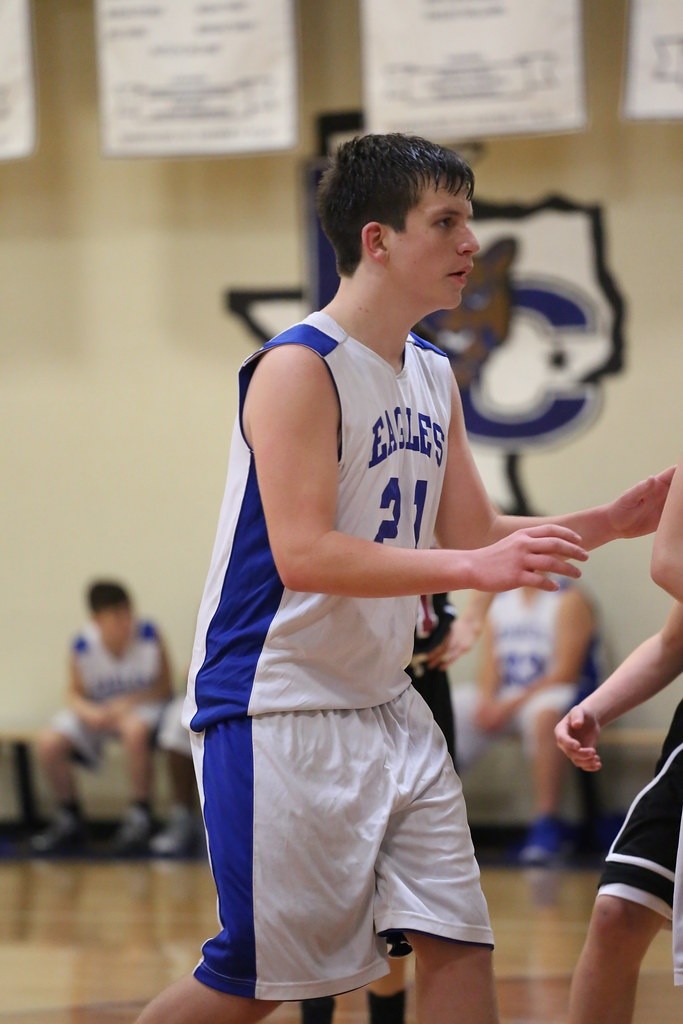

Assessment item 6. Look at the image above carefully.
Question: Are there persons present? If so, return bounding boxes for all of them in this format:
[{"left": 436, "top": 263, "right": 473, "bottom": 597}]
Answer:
[
  {"left": 553, "top": 463, "right": 683, "bottom": 1023},
  {"left": 30, "top": 583, "right": 174, "bottom": 854},
  {"left": 451, "top": 573, "right": 599, "bottom": 867},
  {"left": 301, "top": 587, "right": 495, "bottom": 1023},
  {"left": 146, "top": 697, "right": 205, "bottom": 854},
  {"left": 133, "top": 133, "right": 678, "bottom": 1024}
]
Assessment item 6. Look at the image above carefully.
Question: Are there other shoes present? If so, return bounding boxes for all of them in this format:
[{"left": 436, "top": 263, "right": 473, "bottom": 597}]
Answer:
[
  {"left": 521, "top": 814, "right": 564, "bottom": 866},
  {"left": 113, "top": 801, "right": 153, "bottom": 850},
  {"left": 147, "top": 813, "right": 202, "bottom": 855},
  {"left": 31, "top": 803, "right": 92, "bottom": 853}
]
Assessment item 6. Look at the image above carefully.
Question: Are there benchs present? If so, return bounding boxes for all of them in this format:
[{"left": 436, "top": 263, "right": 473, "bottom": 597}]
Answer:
[{"left": 0, "top": 727, "right": 666, "bottom": 874}]
